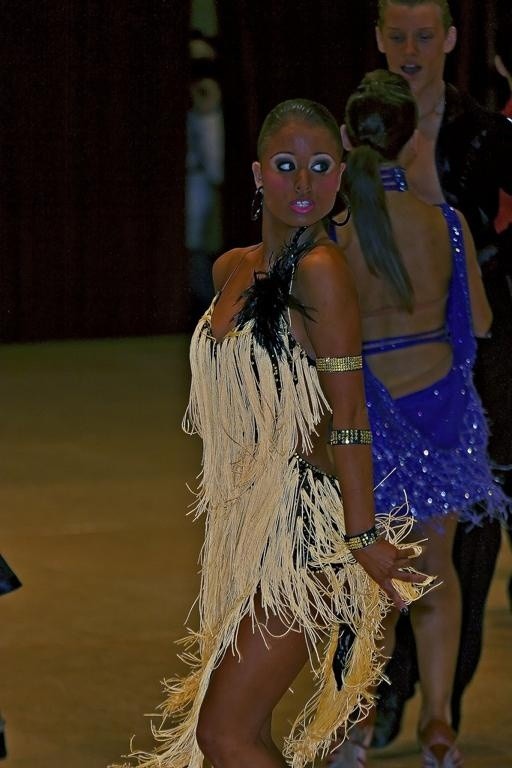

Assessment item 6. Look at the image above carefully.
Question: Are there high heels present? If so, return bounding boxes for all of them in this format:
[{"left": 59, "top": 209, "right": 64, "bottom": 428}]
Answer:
[{"left": 416, "top": 716, "right": 465, "bottom": 768}]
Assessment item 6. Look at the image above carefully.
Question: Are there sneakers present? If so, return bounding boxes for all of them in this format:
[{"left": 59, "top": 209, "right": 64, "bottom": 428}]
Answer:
[{"left": 324, "top": 705, "right": 377, "bottom": 768}]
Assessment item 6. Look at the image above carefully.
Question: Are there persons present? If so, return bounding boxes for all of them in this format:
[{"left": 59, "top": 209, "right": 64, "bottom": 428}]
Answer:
[
  {"left": 322, "top": 68, "right": 512, "bottom": 768},
  {"left": 107, "top": 96, "right": 446, "bottom": 768},
  {"left": 367, "top": 0, "right": 512, "bottom": 751}
]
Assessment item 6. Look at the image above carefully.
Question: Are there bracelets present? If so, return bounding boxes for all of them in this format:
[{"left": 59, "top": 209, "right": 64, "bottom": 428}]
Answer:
[{"left": 343, "top": 528, "right": 378, "bottom": 552}]
